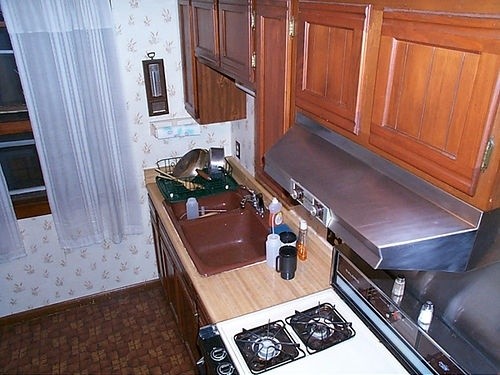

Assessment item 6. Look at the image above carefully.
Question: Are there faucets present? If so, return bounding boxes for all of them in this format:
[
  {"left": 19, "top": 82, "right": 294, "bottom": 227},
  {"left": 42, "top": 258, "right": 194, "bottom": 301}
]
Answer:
[{"left": 240, "top": 186, "right": 265, "bottom": 215}]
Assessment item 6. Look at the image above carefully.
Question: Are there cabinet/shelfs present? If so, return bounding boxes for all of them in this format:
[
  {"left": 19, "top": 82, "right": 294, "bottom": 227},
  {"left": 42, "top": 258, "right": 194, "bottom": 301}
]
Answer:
[
  {"left": 146, "top": 182, "right": 331, "bottom": 371},
  {"left": 291, "top": 0, "right": 499, "bottom": 211},
  {"left": 254, "top": 0, "right": 295, "bottom": 211},
  {"left": 177, "top": 0, "right": 246, "bottom": 125},
  {"left": 189, "top": 1, "right": 255, "bottom": 87}
]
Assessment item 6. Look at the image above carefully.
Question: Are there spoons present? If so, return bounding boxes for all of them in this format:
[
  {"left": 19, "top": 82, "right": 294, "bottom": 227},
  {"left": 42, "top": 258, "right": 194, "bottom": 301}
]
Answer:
[{"left": 156, "top": 169, "right": 203, "bottom": 191}]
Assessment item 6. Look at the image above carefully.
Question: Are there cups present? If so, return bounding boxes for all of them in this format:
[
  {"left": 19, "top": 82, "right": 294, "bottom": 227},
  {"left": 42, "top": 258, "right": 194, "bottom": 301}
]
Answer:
[{"left": 276, "top": 246, "right": 297, "bottom": 280}]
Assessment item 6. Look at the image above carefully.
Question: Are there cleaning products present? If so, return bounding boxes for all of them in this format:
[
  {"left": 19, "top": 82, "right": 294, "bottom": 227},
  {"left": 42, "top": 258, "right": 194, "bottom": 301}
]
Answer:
[
  {"left": 298, "top": 221, "right": 308, "bottom": 261},
  {"left": 269, "top": 197, "right": 284, "bottom": 224}
]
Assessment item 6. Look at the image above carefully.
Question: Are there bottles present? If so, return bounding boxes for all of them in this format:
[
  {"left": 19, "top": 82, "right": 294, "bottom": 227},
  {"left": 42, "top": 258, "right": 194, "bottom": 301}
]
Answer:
[
  {"left": 268, "top": 197, "right": 284, "bottom": 228},
  {"left": 417, "top": 301, "right": 435, "bottom": 326},
  {"left": 265, "top": 234, "right": 281, "bottom": 269},
  {"left": 186, "top": 197, "right": 199, "bottom": 220},
  {"left": 296, "top": 220, "right": 308, "bottom": 260},
  {"left": 279, "top": 232, "right": 297, "bottom": 250},
  {"left": 391, "top": 274, "right": 406, "bottom": 296}
]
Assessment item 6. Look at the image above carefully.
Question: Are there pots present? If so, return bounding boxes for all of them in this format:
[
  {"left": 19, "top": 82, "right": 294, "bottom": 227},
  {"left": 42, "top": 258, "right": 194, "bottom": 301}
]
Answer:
[
  {"left": 208, "top": 147, "right": 227, "bottom": 179},
  {"left": 173, "top": 149, "right": 212, "bottom": 184}
]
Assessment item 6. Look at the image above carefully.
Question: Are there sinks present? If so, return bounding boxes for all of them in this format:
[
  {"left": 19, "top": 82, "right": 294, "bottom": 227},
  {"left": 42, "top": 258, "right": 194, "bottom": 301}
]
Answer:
[
  {"left": 161, "top": 185, "right": 255, "bottom": 222},
  {"left": 181, "top": 211, "right": 281, "bottom": 275}
]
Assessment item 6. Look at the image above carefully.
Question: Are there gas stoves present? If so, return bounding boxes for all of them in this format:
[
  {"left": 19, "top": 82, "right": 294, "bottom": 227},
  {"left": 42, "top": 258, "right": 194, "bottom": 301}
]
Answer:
[{"left": 200, "top": 288, "right": 412, "bottom": 375}]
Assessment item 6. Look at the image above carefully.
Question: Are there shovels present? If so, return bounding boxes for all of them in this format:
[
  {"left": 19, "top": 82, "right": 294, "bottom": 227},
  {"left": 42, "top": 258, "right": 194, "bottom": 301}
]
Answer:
[{"left": 156, "top": 168, "right": 204, "bottom": 191}]
